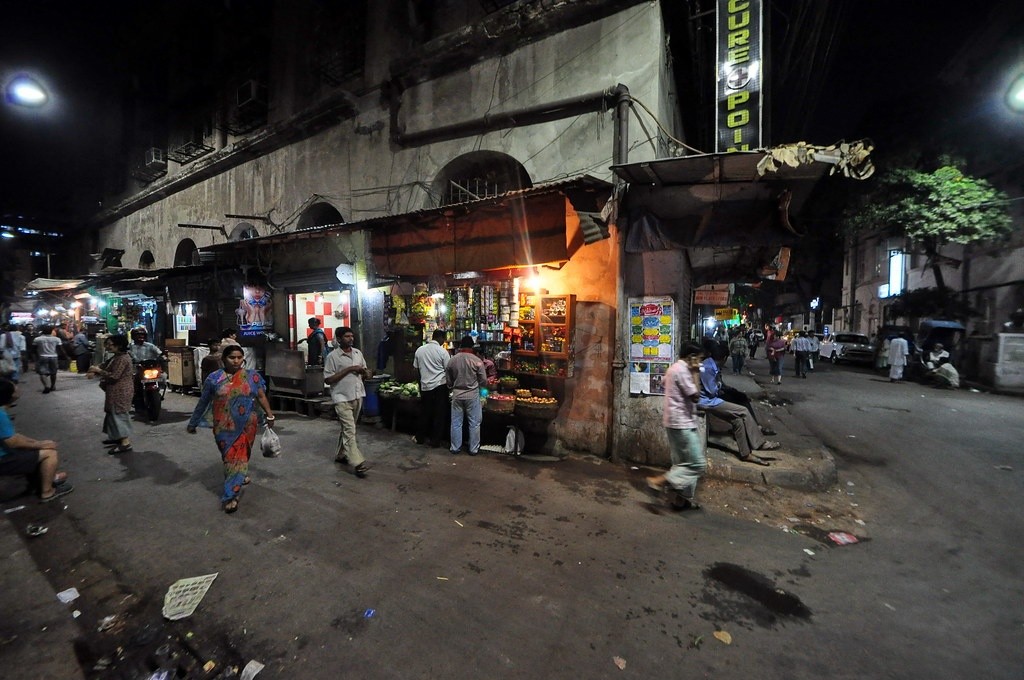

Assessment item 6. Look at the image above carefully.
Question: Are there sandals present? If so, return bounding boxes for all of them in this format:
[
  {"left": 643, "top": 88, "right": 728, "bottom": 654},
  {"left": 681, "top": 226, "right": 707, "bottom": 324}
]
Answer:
[
  {"left": 224, "top": 495, "right": 240, "bottom": 513},
  {"left": 108, "top": 443, "right": 132, "bottom": 454}
]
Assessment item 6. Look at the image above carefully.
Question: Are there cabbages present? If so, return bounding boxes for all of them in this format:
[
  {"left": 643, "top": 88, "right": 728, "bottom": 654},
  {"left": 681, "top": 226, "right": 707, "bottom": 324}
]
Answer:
[{"left": 402, "top": 382, "right": 421, "bottom": 396}]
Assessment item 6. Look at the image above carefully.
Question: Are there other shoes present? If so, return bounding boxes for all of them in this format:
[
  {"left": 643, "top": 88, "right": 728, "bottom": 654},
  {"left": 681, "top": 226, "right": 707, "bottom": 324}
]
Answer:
[
  {"left": 53, "top": 471, "right": 68, "bottom": 483},
  {"left": 746, "top": 454, "right": 770, "bottom": 466},
  {"left": 336, "top": 456, "right": 349, "bottom": 465},
  {"left": 673, "top": 499, "right": 702, "bottom": 511},
  {"left": 356, "top": 464, "right": 373, "bottom": 474},
  {"left": 757, "top": 441, "right": 780, "bottom": 451},
  {"left": 103, "top": 437, "right": 121, "bottom": 444},
  {"left": 647, "top": 477, "right": 661, "bottom": 499},
  {"left": 42, "top": 483, "right": 73, "bottom": 501},
  {"left": 762, "top": 428, "right": 776, "bottom": 435}
]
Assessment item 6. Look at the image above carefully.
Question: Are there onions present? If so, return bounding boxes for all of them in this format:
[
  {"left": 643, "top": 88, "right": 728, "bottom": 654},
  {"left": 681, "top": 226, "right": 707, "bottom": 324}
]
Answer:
[{"left": 489, "top": 389, "right": 556, "bottom": 403}]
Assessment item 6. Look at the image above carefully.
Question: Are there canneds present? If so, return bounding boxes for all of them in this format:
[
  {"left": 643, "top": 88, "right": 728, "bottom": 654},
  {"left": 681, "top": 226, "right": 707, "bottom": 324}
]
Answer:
[
  {"left": 481, "top": 331, "right": 486, "bottom": 341},
  {"left": 486, "top": 332, "right": 503, "bottom": 341},
  {"left": 524, "top": 341, "right": 528, "bottom": 350}
]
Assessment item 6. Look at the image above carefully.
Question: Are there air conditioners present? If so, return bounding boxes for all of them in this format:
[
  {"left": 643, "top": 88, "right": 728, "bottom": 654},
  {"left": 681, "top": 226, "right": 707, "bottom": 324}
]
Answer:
[
  {"left": 145, "top": 147, "right": 167, "bottom": 170},
  {"left": 235, "top": 80, "right": 257, "bottom": 107}
]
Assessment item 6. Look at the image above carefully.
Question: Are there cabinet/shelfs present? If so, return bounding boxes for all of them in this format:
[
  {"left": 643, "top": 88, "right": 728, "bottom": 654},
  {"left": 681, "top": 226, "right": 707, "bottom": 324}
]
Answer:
[{"left": 512, "top": 293, "right": 576, "bottom": 378}]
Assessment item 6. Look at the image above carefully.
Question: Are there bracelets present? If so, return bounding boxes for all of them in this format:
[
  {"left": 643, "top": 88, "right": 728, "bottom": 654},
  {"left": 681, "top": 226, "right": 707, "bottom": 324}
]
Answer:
[{"left": 267, "top": 416, "right": 274, "bottom": 420}]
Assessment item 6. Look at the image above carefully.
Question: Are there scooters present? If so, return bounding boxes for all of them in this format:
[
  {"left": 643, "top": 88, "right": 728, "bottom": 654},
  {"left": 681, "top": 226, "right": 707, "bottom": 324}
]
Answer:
[{"left": 130, "top": 348, "right": 182, "bottom": 417}]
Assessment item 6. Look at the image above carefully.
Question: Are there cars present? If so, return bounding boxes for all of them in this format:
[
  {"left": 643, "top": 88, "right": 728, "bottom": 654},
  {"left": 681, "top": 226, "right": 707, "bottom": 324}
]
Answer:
[
  {"left": 754, "top": 329, "right": 769, "bottom": 340},
  {"left": 782, "top": 328, "right": 826, "bottom": 353},
  {"left": 819, "top": 332, "right": 878, "bottom": 366}
]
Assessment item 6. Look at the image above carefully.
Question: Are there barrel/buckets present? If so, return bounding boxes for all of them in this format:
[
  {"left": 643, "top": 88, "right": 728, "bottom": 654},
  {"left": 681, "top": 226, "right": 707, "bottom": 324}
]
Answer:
[{"left": 363, "top": 378, "right": 382, "bottom": 416}]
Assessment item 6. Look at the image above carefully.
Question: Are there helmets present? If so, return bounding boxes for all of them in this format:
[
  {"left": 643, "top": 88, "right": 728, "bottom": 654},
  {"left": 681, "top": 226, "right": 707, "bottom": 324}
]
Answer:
[{"left": 131, "top": 325, "right": 148, "bottom": 339}]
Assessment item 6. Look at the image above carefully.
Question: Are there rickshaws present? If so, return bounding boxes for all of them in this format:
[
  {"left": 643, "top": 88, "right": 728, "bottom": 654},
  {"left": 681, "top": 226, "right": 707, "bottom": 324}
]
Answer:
[{"left": 875, "top": 320, "right": 970, "bottom": 387}]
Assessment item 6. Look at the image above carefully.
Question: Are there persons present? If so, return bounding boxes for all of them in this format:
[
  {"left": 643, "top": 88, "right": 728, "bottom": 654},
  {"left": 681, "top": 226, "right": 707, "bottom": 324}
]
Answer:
[
  {"left": 187, "top": 346, "right": 275, "bottom": 512},
  {"left": 32, "top": 325, "right": 64, "bottom": 394},
  {"left": 323, "top": 327, "right": 374, "bottom": 473},
  {"left": 412, "top": 328, "right": 450, "bottom": 447},
  {"left": 929, "top": 343, "right": 959, "bottom": 388},
  {"left": 714, "top": 322, "right": 759, "bottom": 376},
  {"left": 645, "top": 341, "right": 708, "bottom": 511},
  {"left": 307, "top": 317, "right": 329, "bottom": 365},
  {"left": 474, "top": 349, "right": 498, "bottom": 378},
  {"left": 882, "top": 330, "right": 909, "bottom": 382},
  {"left": 216, "top": 328, "right": 242, "bottom": 352},
  {"left": 128, "top": 326, "right": 167, "bottom": 373},
  {"left": 0, "top": 378, "right": 75, "bottom": 502},
  {"left": 0, "top": 323, "right": 94, "bottom": 383},
  {"left": 763, "top": 323, "right": 820, "bottom": 384},
  {"left": 201, "top": 337, "right": 223, "bottom": 384},
  {"left": 699, "top": 340, "right": 782, "bottom": 466},
  {"left": 85, "top": 334, "right": 135, "bottom": 455},
  {"left": 445, "top": 335, "right": 488, "bottom": 456}
]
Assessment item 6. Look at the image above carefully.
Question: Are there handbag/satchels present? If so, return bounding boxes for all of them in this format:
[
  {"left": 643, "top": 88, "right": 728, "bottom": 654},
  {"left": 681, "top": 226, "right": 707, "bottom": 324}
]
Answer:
[{"left": 260, "top": 425, "right": 281, "bottom": 458}]
